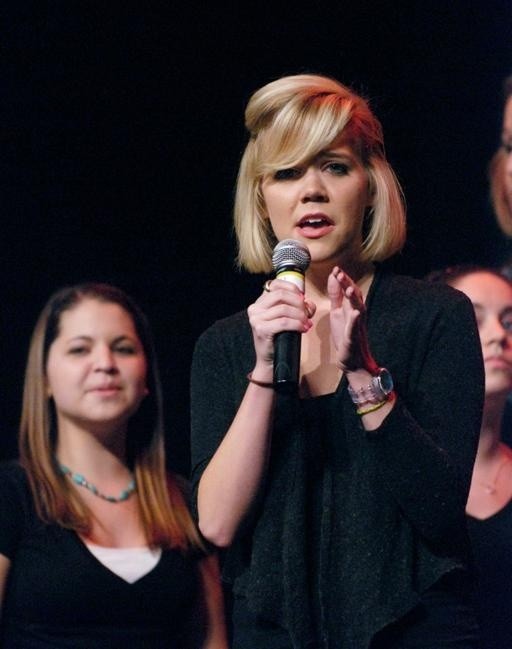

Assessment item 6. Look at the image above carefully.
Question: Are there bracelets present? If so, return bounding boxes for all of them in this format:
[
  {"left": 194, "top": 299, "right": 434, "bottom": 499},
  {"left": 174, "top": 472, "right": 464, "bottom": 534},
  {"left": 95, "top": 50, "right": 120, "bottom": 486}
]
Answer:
[
  {"left": 355, "top": 392, "right": 386, "bottom": 415},
  {"left": 244, "top": 371, "right": 274, "bottom": 389}
]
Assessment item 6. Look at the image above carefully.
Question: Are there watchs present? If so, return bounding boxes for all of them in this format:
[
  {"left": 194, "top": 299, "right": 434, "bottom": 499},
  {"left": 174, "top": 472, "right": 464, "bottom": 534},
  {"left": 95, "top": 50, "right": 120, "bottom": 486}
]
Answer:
[{"left": 346, "top": 366, "right": 394, "bottom": 404}]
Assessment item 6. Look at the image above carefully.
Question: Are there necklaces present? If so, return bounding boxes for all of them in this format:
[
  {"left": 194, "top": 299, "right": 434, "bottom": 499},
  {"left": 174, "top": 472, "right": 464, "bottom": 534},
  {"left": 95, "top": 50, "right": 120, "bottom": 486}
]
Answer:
[{"left": 57, "top": 459, "right": 138, "bottom": 503}]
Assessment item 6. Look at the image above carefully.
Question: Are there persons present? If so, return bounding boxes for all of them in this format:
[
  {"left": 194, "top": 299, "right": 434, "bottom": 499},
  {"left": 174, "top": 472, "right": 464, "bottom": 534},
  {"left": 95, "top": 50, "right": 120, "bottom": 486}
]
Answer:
[
  {"left": 487, "top": 90, "right": 511, "bottom": 237},
  {"left": 188, "top": 69, "right": 486, "bottom": 647},
  {"left": 1, "top": 279, "right": 227, "bottom": 649},
  {"left": 418, "top": 253, "right": 511, "bottom": 649}
]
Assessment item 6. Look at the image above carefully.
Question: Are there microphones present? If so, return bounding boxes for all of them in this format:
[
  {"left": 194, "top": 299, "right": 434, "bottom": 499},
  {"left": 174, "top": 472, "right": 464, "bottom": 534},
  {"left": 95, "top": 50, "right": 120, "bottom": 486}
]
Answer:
[{"left": 271, "top": 239, "right": 312, "bottom": 388}]
